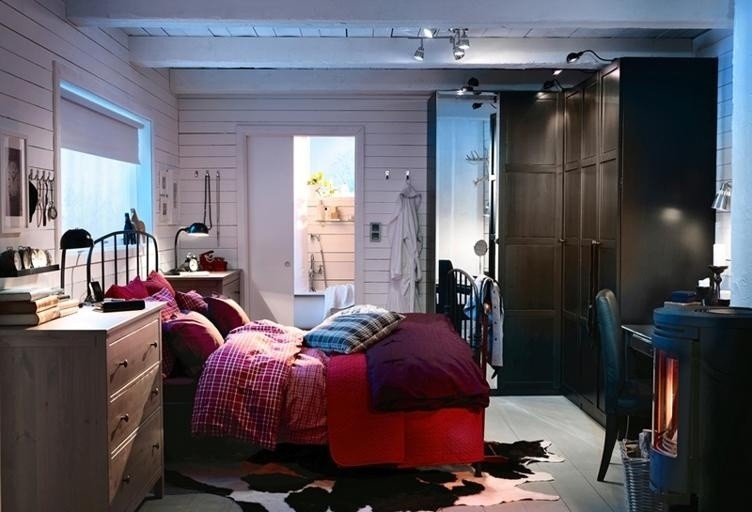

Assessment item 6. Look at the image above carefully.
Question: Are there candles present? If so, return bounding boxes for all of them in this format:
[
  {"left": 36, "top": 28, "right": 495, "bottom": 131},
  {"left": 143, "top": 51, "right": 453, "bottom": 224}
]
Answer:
[
  {"left": 698, "top": 278, "right": 710, "bottom": 286},
  {"left": 712, "top": 244, "right": 724, "bottom": 265}
]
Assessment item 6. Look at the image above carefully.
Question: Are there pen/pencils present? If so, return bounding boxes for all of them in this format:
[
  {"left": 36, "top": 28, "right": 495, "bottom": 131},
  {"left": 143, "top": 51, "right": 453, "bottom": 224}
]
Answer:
[{"left": 104, "top": 299, "right": 125, "bottom": 301}]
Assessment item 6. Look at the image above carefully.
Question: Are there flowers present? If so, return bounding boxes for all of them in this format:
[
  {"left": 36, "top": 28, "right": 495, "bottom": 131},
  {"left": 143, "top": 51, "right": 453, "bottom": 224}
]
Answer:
[{"left": 307, "top": 172, "right": 335, "bottom": 200}]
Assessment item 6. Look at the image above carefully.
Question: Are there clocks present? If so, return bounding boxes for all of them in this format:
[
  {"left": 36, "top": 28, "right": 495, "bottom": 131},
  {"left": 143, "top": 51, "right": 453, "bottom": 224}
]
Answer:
[{"left": 185, "top": 252, "right": 199, "bottom": 272}]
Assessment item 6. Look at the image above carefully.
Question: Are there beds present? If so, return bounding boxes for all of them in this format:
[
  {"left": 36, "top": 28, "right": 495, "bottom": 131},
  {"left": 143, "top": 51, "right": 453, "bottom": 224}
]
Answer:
[{"left": 86, "top": 228, "right": 482, "bottom": 478}]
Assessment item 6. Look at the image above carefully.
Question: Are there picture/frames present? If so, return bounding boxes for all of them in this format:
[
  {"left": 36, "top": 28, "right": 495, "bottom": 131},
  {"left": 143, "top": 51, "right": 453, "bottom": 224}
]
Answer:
[
  {"left": 159, "top": 170, "right": 169, "bottom": 196},
  {"left": 160, "top": 198, "right": 170, "bottom": 222},
  {"left": 0, "top": 133, "right": 28, "bottom": 236}
]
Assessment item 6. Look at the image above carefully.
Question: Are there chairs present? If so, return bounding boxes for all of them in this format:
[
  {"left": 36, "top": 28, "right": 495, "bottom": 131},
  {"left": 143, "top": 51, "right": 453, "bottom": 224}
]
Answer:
[{"left": 594, "top": 289, "right": 652, "bottom": 482}]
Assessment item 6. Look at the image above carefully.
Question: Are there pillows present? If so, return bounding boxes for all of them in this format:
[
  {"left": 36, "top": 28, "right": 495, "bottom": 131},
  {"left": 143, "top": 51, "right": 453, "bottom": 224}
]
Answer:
[
  {"left": 163, "top": 310, "right": 226, "bottom": 374},
  {"left": 144, "top": 287, "right": 180, "bottom": 321},
  {"left": 178, "top": 288, "right": 207, "bottom": 312},
  {"left": 107, "top": 275, "right": 149, "bottom": 300},
  {"left": 142, "top": 270, "right": 177, "bottom": 298},
  {"left": 302, "top": 304, "right": 406, "bottom": 356},
  {"left": 205, "top": 290, "right": 251, "bottom": 337}
]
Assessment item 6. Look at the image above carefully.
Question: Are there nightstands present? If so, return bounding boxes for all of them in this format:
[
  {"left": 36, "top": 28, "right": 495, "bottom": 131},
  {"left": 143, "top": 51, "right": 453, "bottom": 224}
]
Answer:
[{"left": 0, "top": 302, "right": 169, "bottom": 512}]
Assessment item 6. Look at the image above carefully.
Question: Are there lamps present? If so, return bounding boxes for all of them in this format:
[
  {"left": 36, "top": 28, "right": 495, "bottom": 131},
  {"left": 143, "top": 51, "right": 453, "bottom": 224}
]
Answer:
[
  {"left": 711, "top": 181, "right": 731, "bottom": 212},
  {"left": 168, "top": 223, "right": 209, "bottom": 275},
  {"left": 60, "top": 228, "right": 94, "bottom": 288},
  {"left": 414, "top": 26, "right": 616, "bottom": 110}
]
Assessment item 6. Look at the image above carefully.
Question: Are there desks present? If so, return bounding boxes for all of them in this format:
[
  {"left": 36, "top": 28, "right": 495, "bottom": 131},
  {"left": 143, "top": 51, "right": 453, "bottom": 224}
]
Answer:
[{"left": 622, "top": 324, "right": 652, "bottom": 437}]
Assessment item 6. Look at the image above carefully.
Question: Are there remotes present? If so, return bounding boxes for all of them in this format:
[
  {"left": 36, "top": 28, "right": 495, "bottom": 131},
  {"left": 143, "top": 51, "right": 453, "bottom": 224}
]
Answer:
[{"left": 102, "top": 299, "right": 146, "bottom": 311}]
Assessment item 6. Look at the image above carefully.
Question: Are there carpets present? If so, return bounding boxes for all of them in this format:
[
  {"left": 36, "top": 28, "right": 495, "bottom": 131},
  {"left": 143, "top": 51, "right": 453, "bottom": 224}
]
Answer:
[{"left": 162, "top": 439, "right": 567, "bottom": 512}]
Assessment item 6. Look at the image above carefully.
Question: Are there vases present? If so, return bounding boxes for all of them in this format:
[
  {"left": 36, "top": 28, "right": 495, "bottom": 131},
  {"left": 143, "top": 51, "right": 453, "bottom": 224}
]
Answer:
[{"left": 319, "top": 202, "right": 326, "bottom": 220}]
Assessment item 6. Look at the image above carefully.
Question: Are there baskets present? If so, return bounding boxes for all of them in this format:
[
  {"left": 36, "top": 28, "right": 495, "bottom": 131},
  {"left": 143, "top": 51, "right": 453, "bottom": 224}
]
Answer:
[{"left": 621, "top": 439, "right": 664, "bottom": 512}]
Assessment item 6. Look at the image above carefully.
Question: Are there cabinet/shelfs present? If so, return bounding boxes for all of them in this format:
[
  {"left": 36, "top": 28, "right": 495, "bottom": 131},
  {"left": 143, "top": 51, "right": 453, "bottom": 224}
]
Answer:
[{"left": 498, "top": 57, "right": 718, "bottom": 434}]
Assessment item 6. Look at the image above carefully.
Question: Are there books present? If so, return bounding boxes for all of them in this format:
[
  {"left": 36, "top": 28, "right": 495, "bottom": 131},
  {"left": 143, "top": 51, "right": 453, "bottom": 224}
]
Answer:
[{"left": 0, "top": 287, "right": 79, "bottom": 327}]
